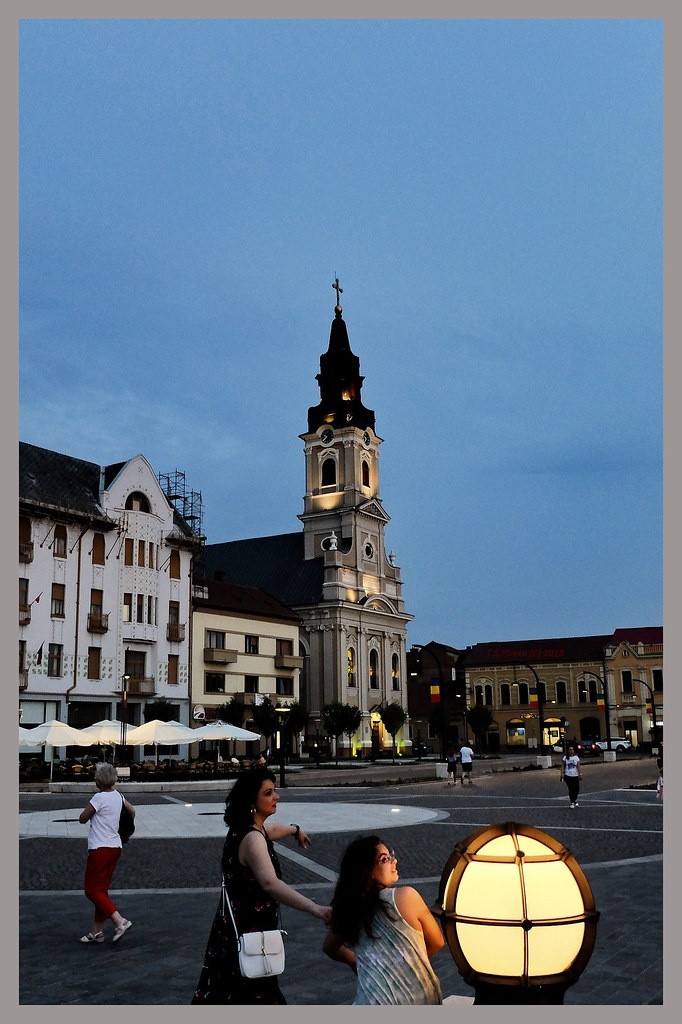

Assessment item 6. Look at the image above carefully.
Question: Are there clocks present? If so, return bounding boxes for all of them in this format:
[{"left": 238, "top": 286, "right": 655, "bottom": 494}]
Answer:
[
  {"left": 363, "top": 432, "right": 370, "bottom": 446},
  {"left": 321, "top": 429, "right": 335, "bottom": 444}
]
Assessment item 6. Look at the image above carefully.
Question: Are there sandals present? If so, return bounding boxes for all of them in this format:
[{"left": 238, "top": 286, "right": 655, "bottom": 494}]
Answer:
[
  {"left": 80, "top": 931, "right": 105, "bottom": 942},
  {"left": 113, "top": 918, "right": 132, "bottom": 941}
]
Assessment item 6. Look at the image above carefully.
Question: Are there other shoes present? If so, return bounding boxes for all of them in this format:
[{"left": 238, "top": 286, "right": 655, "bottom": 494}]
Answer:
[
  {"left": 448, "top": 781, "right": 451, "bottom": 785},
  {"left": 454, "top": 781, "right": 458, "bottom": 784},
  {"left": 469, "top": 781, "right": 473, "bottom": 784},
  {"left": 461, "top": 778, "right": 464, "bottom": 784},
  {"left": 570, "top": 802, "right": 579, "bottom": 808}
]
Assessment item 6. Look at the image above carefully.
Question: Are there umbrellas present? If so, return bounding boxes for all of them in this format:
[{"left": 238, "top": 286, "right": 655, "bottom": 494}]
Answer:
[{"left": 19, "top": 719, "right": 262, "bottom": 782}]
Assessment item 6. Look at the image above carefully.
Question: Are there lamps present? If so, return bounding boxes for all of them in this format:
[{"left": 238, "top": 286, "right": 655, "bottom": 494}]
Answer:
[
  {"left": 428, "top": 822, "right": 601, "bottom": 1005},
  {"left": 397, "top": 753, "right": 402, "bottom": 758},
  {"left": 353, "top": 755, "right": 358, "bottom": 759}
]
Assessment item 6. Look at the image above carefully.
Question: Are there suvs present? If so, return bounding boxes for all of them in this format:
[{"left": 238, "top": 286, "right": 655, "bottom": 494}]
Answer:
[{"left": 595, "top": 736, "right": 632, "bottom": 753}]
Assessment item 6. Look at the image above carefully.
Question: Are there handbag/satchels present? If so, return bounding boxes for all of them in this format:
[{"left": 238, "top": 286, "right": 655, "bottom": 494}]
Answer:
[
  {"left": 236, "top": 929, "right": 285, "bottom": 978},
  {"left": 118, "top": 792, "right": 136, "bottom": 844}
]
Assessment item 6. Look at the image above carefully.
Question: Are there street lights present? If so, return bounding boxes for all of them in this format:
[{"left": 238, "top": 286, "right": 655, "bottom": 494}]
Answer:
[
  {"left": 411, "top": 643, "right": 454, "bottom": 778},
  {"left": 275, "top": 707, "right": 291, "bottom": 788},
  {"left": 414, "top": 719, "right": 422, "bottom": 759},
  {"left": 631, "top": 678, "right": 659, "bottom": 756},
  {"left": 122, "top": 674, "right": 131, "bottom": 766},
  {"left": 583, "top": 671, "right": 617, "bottom": 761},
  {"left": 313, "top": 719, "right": 322, "bottom": 764},
  {"left": 511, "top": 658, "right": 552, "bottom": 767}
]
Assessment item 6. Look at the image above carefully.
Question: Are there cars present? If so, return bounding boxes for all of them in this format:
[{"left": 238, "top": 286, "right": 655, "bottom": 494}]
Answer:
[
  {"left": 577, "top": 740, "right": 599, "bottom": 757},
  {"left": 552, "top": 739, "right": 577, "bottom": 752}
]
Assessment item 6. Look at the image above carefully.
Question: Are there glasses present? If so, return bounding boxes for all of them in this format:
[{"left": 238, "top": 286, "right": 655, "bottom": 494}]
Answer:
[{"left": 376, "top": 850, "right": 395, "bottom": 866}]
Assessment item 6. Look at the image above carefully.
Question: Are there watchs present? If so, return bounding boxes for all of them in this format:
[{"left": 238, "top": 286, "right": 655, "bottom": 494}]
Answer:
[{"left": 290, "top": 824, "right": 300, "bottom": 836}]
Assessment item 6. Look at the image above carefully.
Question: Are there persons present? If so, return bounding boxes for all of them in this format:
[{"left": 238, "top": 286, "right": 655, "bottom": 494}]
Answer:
[
  {"left": 446, "top": 740, "right": 474, "bottom": 785},
  {"left": 560, "top": 744, "right": 584, "bottom": 809},
  {"left": 191, "top": 763, "right": 333, "bottom": 1005},
  {"left": 323, "top": 835, "right": 446, "bottom": 1005},
  {"left": 79, "top": 762, "right": 136, "bottom": 943}
]
